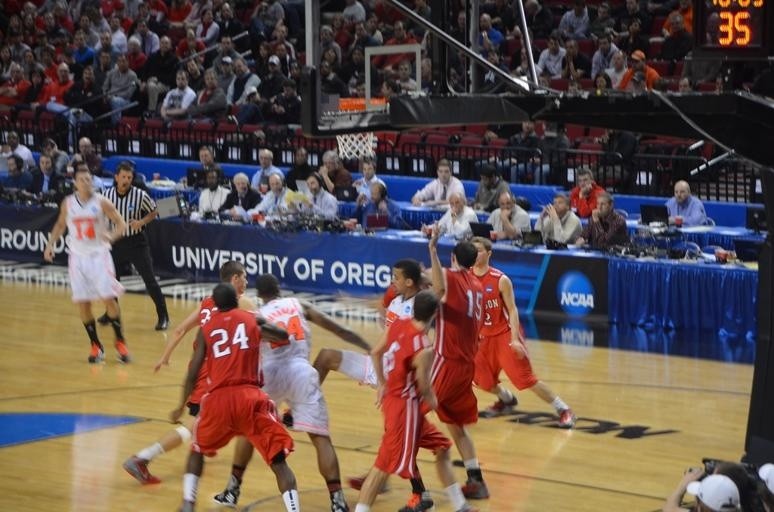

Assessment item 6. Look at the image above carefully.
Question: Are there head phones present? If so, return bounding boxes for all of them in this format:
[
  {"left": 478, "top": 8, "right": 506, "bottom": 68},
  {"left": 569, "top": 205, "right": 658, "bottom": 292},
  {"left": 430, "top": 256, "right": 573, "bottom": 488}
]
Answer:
[{"left": 304, "top": 172, "right": 324, "bottom": 194}]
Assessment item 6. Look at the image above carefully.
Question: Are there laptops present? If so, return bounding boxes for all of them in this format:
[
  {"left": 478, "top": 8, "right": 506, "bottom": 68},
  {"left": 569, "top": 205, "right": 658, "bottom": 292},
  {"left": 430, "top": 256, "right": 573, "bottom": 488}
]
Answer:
[
  {"left": 188, "top": 169, "right": 205, "bottom": 187},
  {"left": 365, "top": 214, "right": 388, "bottom": 233},
  {"left": 640, "top": 205, "right": 669, "bottom": 227},
  {"left": 335, "top": 187, "right": 356, "bottom": 201},
  {"left": 519, "top": 229, "right": 544, "bottom": 245},
  {"left": 733, "top": 240, "right": 765, "bottom": 262},
  {"left": 470, "top": 223, "right": 494, "bottom": 239}
]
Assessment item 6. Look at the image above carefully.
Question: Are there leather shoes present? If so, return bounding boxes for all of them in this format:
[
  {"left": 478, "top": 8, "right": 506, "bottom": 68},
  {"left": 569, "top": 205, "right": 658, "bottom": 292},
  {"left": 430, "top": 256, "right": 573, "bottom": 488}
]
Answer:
[
  {"left": 154, "top": 314, "right": 169, "bottom": 329},
  {"left": 98, "top": 310, "right": 110, "bottom": 325}
]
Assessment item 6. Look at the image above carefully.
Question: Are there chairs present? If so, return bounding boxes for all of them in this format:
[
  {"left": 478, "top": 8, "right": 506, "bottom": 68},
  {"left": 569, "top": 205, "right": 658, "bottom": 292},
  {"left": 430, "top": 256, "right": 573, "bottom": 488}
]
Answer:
[
  {"left": 240, "top": 123, "right": 262, "bottom": 133},
  {"left": 294, "top": 126, "right": 324, "bottom": 148},
  {"left": 408, "top": 126, "right": 436, "bottom": 134},
  {"left": 572, "top": 143, "right": 604, "bottom": 164},
  {"left": 483, "top": 139, "right": 509, "bottom": 157},
  {"left": 644, "top": 39, "right": 671, "bottom": 56},
  {"left": 588, "top": 127, "right": 607, "bottom": 139},
  {"left": 364, "top": 130, "right": 394, "bottom": 151},
  {"left": 675, "top": 60, "right": 686, "bottom": 78},
  {"left": 115, "top": 116, "right": 139, "bottom": 139},
  {"left": 467, "top": 121, "right": 488, "bottom": 136},
  {"left": 0, "top": 110, "right": 15, "bottom": 128},
  {"left": 533, "top": 38, "right": 552, "bottom": 62},
  {"left": 636, "top": 139, "right": 673, "bottom": 156},
  {"left": 395, "top": 133, "right": 423, "bottom": 152},
  {"left": 533, "top": 119, "right": 549, "bottom": 136},
  {"left": 421, "top": 134, "right": 451, "bottom": 158},
  {"left": 576, "top": 79, "right": 595, "bottom": 89},
  {"left": 218, "top": 121, "right": 237, "bottom": 133},
  {"left": 697, "top": 81, "right": 717, "bottom": 92},
  {"left": 666, "top": 82, "right": 680, "bottom": 91},
  {"left": 16, "top": 108, "right": 38, "bottom": 130},
  {"left": 456, "top": 137, "right": 484, "bottom": 158},
  {"left": 576, "top": 38, "right": 598, "bottom": 67},
  {"left": 649, "top": 57, "right": 673, "bottom": 79},
  {"left": 140, "top": 118, "right": 166, "bottom": 142},
  {"left": 672, "top": 141, "right": 713, "bottom": 159},
  {"left": 437, "top": 126, "right": 464, "bottom": 133},
  {"left": 505, "top": 42, "right": 527, "bottom": 58},
  {"left": 167, "top": 121, "right": 191, "bottom": 145},
  {"left": 38, "top": 112, "right": 57, "bottom": 130},
  {"left": 191, "top": 123, "right": 215, "bottom": 144},
  {"left": 565, "top": 119, "right": 586, "bottom": 146},
  {"left": 547, "top": 78, "right": 570, "bottom": 91}
]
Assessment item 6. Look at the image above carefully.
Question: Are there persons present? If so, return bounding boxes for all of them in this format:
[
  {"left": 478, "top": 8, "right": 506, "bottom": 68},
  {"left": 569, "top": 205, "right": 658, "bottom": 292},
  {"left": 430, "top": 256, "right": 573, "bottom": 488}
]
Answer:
[
  {"left": 22, "top": 157, "right": 63, "bottom": 202},
  {"left": 664, "top": 457, "right": 772, "bottom": 512},
  {"left": 660, "top": 2, "right": 695, "bottom": 37},
  {"left": 533, "top": 193, "right": 583, "bottom": 244},
  {"left": 122, "top": 36, "right": 146, "bottom": 70},
  {"left": 481, "top": 50, "right": 508, "bottom": 86},
  {"left": 3, "top": 0, "right": 75, "bottom": 37},
  {"left": 631, "top": 71, "right": 645, "bottom": 92},
  {"left": 447, "top": 237, "right": 573, "bottom": 427},
  {"left": 526, "top": 0, "right": 552, "bottom": 35},
  {"left": 275, "top": 42, "right": 293, "bottom": 80},
  {"left": 129, "top": 21, "right": 157, "bottom": 52},
  {"left": 470, "top": 164, "right": 510, "bottom": 211},
  {"left": 481, "top": 191, "right": 530, "bottom": 240},
  {"left": 340, "top": 47, "right": 375, "bottom": 93},
  {"left": 391, "top": 59, "right": 416, "bottom": 92},
  {"left": 616, "top": 0, "right": 650, "bottom": 28},
  {"left": 1, "top": 132, "right": 32, "bottom": 173},
  {"left": 660, "top": 15, "right": 691, "bottom": 61},
  {"left": 448, "top": 10, "right": 467, "bottom": 47},
  {"left": 318, "top": 49, "right": 338, "bottom": 68},
  {"left": 184, "top": 57, "right": 205, "bottom": 89},
  {"left": 20, "top": 50, "right": 44, "bottom": 78},
  {"left": 591, "top": 34, "right": 619, "bottom": 84},
  {"left": 617, "top": 50, "right": 658, "bottom": 87},
  {"left": 347, "top": 21, "right": 378, "bottom": 49},
  {"left": 321, "top": 61, "right": 348, "bottom": 96},
  {"left": 74, "top": 14, "right": 99, "bottom": 49},
  {"left": 537, "top": 35, "right": 566, "bottom": 80},
  {"left": 192, "top": 9, "right": 217, "bottom": 48},
  {"left": 412, "top": 160, "right": 467, "bottom": 206},
  {"left": 68, "top": 136, "right": 103, "bottom": 174},
  {"left": 281, "top": 223, "right": 445, "bottom": 512},
  {"left": 124, "top": 259, "right": 256, "bottom": 487},
  {"left": 189, "top": 70, "right": 225, "bottom": 125},
  {"left": 197, "top": 170, "right": 228, "bottom": 221},
  {"left": 251, "top": 149, "right": 285, "bottom": 192},
  {"left": 576, "top": 193, "right": 627, "bottom": 247},
  {"left": 41, "top": 139, "right": 68, "bottom": 176},
  {"left": 264, "top": 23, "right": 295, "bottom": 78},
  {"left": 363, "top": 19, "right": 383, "bottom": 47},
  {"left": 176, "top": 28, "right": 206, "bottom": 72},
  {"left": 316, "top": 26, "right": 342, "bottom": 63},
  {"left": 246, "top": 172, "right": 296, "bottom": 227},
  {"left": 1, "top": 64, "right": 29, "bottom": 125},
  {"left": 178, "top": 145, "right": 220, "bottom": 189},
  {"left": 67, "top": 30, "right": 96, "bottom": 73},
  {"left": 216, "top": 4, "right": 244, "bottom": 36},
  {"left": 261, "top": 55, "right": 285, "bottom": 100},
  {"left": 508, "top": 122, "right": 568, "bottom": 187},
  {"left": 378, "top": 81, "right": 404, "bottom": 122},
  {"left": 561, "top": 170, "right": 607, "bottom": 217},
  {"left": 31, "top": 31, "right": 53, "bottom": 55},
  {"left": 96, "top": 32, "right": 121, "bottom": 61},
  {"left": 350, "top": 160, "right": 385, "bottom": 201},
  {"left": 285, "top": 174, "right": 337, "bottom": 221},
  {"left": 0, "top": 157, "right": 32, "bottom": 213},
  {"left": 326, "top": 13, "right": 351, "bottom": 51},
  {"left": 373, "top": 20, "right": 420, "bottom": 72},
  {"left": 352, "top": 179, "right": 388, "bottom": 227},
  {"left": 315, "top": 151, "right": 353, "bottom": 200},
  {"left": 217, "top": 172, "right": 260, "bottom": 225},
  {"left": 474, "top": 13, "right": 504, "bottom": 47},
  {"left": 562, "top": 40, "right": 590, "bottom": 82},
  {"left": 423, "top": 192, "right": 477, "bottom": 238},
  {"left": 44, "top": 164, "right": 128, "bottom": 364},
  {"left": 421, "top": 58, "right": 431, "bottom": 88},
  {"left": 566, "top": 82, "right": 579, "bottom": 89},
  {"left": 224, "top": 57, "right": 262, "bottom": 128},
  {"left": 213, "top": 272, "right": 375, "bottom": 510},
  {"left": 137, "top": 36, "right": 180, "bottom": 124},
  {"left": 576, "top": 128, "right": 633, "bottom": 187},
  {"left": 43, "top": 63, "right": 72, "bottom": 123},
  {"left": 160, "top": 69, "right": 196, "bottom": 123},
  {"left": 212, "top": 36, "right": 243, "bottom": 73},
  {"left": 602, "top": 52, "right": 627, "bottom": 84},
  {"left": 63, "top": 66, "right": 103, "bottom": 153},
  {"left": 515, "top": 47, "right": 541, "bottom": 87},
  {"left": 651, "top": 180, "right": 709, "bottom": 226},
  {"left": 583, "top": 3, "right": 616, "bottom": 36},
  {"left": 675, "top": 73, "right": 701, "bottom": 94},
  {"left": 348, "top": 241, "right": 489, "bottom": 499},
  {"left": 252, "top": 0, "right": 283, "bottom": 45},
  {"left": 170, "top": 1, "right": 239, "bottom": 17},
  {"left": 267, "top": 79, "right": 301, "bottom": 124},
  {"left": 105, "top": 16, "right": 130, "bottom": 55},
  {"left": 226, "top": 86, "right": 264, "bottom": 126},
  {"left": 71, "top": 0, "right": 134, "bottom": 24},
  {"left": 331, "top": 0, "right": 394, "bottom": 34},
  {"left": 129, "top": 2, "right": 163, "bottom": 39},
  {"left": 289, "top": 147, "right": 313, "bottom": 187},
  {"left": 0, "top": 29, "right": 36, "bottom": 57},
  {"left": 353, "top": 293, "right": 476, "bottom": 510},
  {"left": 484, "top": 122, "right": 535, "bottom": 180},
  {"left": 169, "top": 280, "right": 299, "bottom": 511},
  {"left": 557, "top": 1, "right": 587, "bottom": 38},
  {"left": 596, "top": 73, "right": 612, "bottom": 89},
  {"left": 217, "top": 56, "right": 236, "bottom": 93},
  {"left": 253, "top": 41, "right": 272, "bottom": 78},
  {"left": 0, "top": 45, "right": 15, "bottom": 76},
  {"left": 101, "top": 56, "right": 137, "bottom": 127},
  {"left": 617, "top": 18, "right": 647, "bottom": 56},
  {"left": 90, "top": 162, "right": 168, "bottom": 329},
  {"left": 712, "top": 71, "right": 722, "bottom": 93}
]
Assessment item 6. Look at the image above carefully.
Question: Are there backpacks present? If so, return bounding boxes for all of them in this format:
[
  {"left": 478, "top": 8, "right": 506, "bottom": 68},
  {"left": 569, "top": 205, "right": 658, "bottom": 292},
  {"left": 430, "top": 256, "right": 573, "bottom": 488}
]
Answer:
[
  {"left": 9, "top": 63, "right": 52, "bottom": 125},
  {"left": 39, "top": 47, "right": 58, "bottom": 75}
]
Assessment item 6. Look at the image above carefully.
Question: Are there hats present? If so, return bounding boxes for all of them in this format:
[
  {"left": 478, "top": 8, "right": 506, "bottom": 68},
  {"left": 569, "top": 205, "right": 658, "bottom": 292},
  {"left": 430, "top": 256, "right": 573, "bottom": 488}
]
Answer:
[
  {"left": 221, "top": 56, "right": 232, "bottom": 65},
  {"left": 686, "top": 474, "right": 740, "bottom": 512},
  {"left": 631, "top": 50, "right": 646, "bottom": 60},
  {"left": 267, "top": 55, "right": 280, "bottom": 65}
]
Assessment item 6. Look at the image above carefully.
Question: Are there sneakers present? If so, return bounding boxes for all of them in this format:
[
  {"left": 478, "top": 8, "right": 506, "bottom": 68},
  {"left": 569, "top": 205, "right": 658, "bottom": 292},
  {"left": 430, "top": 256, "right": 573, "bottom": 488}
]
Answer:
[
  {"left": 212, "top": 488, "right": 238, "bottom": 508},
  {"left": 282, "top": 409, "right": 302, "bottom": 432},
  {"left": 114, "top": 337, "right": 130, "bottom": 363},
  {"left": 122, "top": 455, "right": 160, "bottom": 484},
  {"left": 88, "top": 338, "right": 105, "bottom": 363},
  {"left": 487, "top": 395, "right": 517, "bottom": 417},
  {"left": 461, "top": 480, "right": 490, "bottom": 499},
  {"left": 557, "top": 407, "right": 577, "bottom": 428},
  {"left": 399, "top": 490, "right": 434, "bottom": 511},
  {"left": 349, "top": 476, "right": 387, "bottom": 495}
]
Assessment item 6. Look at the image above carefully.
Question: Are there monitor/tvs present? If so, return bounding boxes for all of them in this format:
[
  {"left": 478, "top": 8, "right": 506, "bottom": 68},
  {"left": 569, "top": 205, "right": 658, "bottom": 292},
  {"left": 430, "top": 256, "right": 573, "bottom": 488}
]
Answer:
[{"left": 746, "top": 208, "right": 767, "bottom": 236}]
